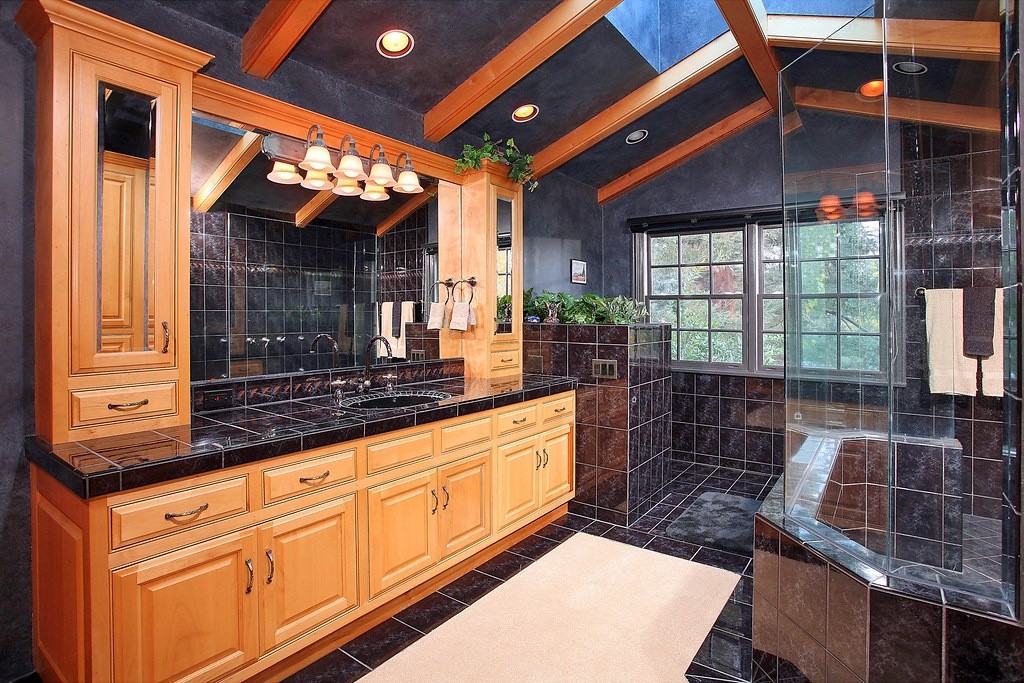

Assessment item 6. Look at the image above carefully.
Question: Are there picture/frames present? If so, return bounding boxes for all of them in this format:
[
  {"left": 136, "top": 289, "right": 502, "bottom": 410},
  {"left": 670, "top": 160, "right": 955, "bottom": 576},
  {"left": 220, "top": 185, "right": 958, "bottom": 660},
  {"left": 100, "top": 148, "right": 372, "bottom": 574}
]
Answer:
[{"left": 570, "top": 259, "right": 587, "bottom": 285}]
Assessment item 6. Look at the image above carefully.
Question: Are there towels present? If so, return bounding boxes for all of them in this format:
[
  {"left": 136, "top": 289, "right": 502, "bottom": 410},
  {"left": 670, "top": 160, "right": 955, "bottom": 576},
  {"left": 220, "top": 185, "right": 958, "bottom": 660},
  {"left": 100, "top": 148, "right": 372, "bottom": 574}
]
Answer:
[
  {"left": 981, "top": 288, "right": 1004, "bottom": 397},
  {"left": 426, "top": 302, "right": 448, "bottom": 330},
  {"left": 449, "top": 302, "right": 477, "bottom": 332},
  {"left": 380, "top": 302, "right": 397, "bottom": 358},
  {"left": 397, "top": 301, "right": 415, "bottom": 359},
  {"left": 392, "top": 301, "right": 402, "bottom": 337},
  {"left": 963, "top": 287, "right": 995, "bottom": 358},
  {"left": 925, "top": 288, "right": 978, "bottom": 398}
]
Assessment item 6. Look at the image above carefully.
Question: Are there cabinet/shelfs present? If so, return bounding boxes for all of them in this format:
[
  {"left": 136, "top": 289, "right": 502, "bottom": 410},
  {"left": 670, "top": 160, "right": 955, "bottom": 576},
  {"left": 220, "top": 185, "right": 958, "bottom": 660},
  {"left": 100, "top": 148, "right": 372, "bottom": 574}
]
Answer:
[
  {"left": 361, "top": 409, "right": 496, "bottom": 632},
  {"left": 463, "top": 172, "right": 523, "bottom": 342},
  {"left": 496, "top": 389, "right": 576, "bottom": 554},
  {"left": 35, "top": 374, "right": 190, "bottom": 445},
  {"left": 35, "top": 24, "right": 191, "bottom": 380},
  {"left": 29, "top": 439, "right": 361, "bottom": 683},
  {"left": 463, "top": 342, "right": 522, "bottom": 379}
]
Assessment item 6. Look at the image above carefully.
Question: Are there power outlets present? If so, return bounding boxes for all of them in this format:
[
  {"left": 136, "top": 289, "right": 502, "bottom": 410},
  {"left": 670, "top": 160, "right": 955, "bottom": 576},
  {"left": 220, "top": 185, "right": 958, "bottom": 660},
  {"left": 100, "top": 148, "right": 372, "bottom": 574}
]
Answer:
[{"left": 592, "top": 359, "right": 617, "bottom": 379}]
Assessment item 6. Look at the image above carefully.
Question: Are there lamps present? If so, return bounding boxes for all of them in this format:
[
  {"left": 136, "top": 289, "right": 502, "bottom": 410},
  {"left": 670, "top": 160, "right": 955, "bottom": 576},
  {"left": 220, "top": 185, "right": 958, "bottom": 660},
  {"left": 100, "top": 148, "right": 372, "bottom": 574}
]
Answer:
[
  {"left": 360, "top": 183, "right": 390, "bottom": 201},
  {"left": 332, "top": 178, "right": 363, "bottom": 196},
  {"left": 297, "top": 124, "right": 336, "bottom": 174},
  {"left": 365, "top": 143, "right": 397, "bottom": 187},
  {"left": 392, "top": 152, "right": 424, "bottom": 193},
  {"left": 301, "top": 170, "right": 334, "bottom": 190},
  {"left": 266, "top": 161, "right": 303, "bottom": 184},
  {"left": 334, "top": 134, "right": 368, "bottom": 181}
]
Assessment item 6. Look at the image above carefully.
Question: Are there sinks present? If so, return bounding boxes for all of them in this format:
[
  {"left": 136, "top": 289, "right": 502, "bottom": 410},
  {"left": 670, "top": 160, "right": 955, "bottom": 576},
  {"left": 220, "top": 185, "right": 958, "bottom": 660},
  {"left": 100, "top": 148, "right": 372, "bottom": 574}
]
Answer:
[{"left": 340, "top": 390, "right": 451, "bottom": 410}]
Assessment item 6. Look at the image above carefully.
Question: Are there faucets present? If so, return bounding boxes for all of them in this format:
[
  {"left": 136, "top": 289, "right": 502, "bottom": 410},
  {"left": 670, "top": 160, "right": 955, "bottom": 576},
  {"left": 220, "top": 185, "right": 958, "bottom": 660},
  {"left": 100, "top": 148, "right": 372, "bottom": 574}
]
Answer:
[
  {"left": 354, "top": 336, "right": 393, "bottom": 394},
  {"left": 309, "top": 333, "right": 339, "bottom": 368}
]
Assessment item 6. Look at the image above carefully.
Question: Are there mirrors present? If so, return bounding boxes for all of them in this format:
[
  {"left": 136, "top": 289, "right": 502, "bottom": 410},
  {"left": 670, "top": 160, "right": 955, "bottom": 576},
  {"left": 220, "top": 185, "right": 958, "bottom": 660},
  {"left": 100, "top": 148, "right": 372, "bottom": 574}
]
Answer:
[
  {"left": 96, "top": 78, "right": 157, "bottom": 354},
  {"left": 495, "top": 194, "right": 513, "bottom": 334},
  {"left": 192, "top": 108, "right": 463, "bottom": 382}
]
